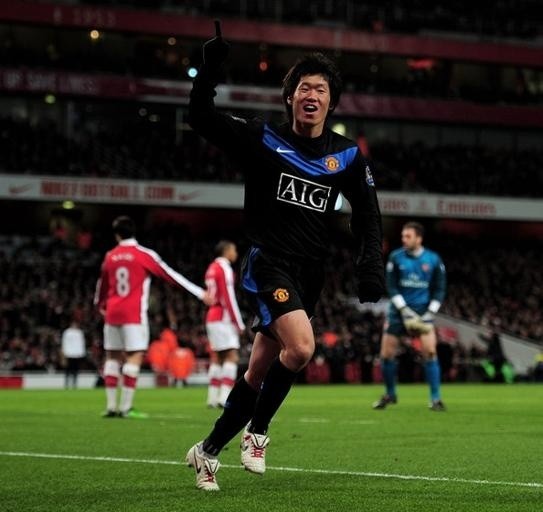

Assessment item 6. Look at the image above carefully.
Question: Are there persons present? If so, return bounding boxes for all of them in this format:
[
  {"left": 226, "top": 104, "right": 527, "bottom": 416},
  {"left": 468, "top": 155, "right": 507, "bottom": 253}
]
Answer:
[
  {"left": 93, "top": 216, "right": 215, "bottom": 417},
  {"left": 372, "top": 221, "right": 446, "bottom": 411},
  {"left": 184, "top": 20, "right": 386, "bottom": 491},
  {"left": 204, "top": 240, "right": 246, "bottom": 409}
]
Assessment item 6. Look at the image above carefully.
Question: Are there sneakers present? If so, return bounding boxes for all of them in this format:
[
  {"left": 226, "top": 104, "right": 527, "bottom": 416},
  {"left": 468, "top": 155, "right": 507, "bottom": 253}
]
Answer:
[
  {"left": 240, "top": 421, "right": 270, "bottom": 474},
  {"left": 103, "top": 409, "right": 128, "bottom": 417},
  {"left": 430, "top": 400, "right": 443, "bottom": 411},
  {"left": 373, "top": 395, "right": 396, "bottom": 408},
  {"left": 185, "top": 441, "right": 220, "bottom": 490}
]
